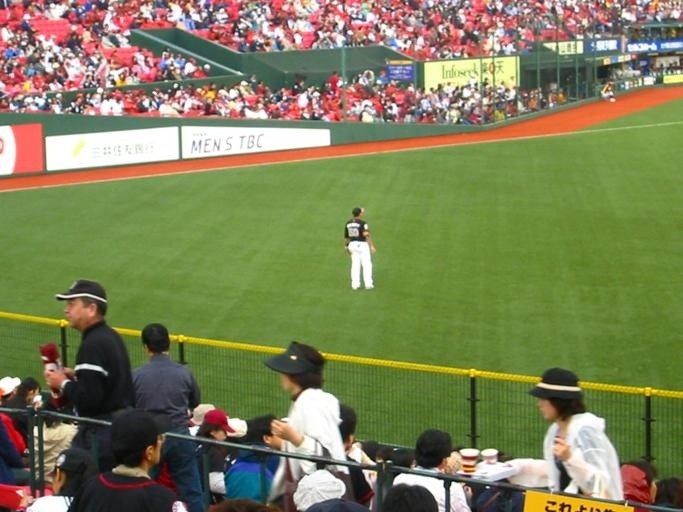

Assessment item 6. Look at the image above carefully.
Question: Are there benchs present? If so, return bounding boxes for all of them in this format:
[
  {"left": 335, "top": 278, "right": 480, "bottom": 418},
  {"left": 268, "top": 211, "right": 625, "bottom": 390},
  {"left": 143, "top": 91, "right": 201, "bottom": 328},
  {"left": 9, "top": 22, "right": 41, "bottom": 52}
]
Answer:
[{"left": 0, "top": 0, "right": 642, "bottom": 127}]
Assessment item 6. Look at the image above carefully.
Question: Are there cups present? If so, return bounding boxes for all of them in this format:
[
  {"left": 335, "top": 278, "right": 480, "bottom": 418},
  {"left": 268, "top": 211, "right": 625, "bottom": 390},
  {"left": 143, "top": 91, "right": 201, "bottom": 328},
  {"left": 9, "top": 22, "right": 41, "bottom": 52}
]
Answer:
[
  {"left": 480, "top": 448, "right": 499, "bottom": 469},
  {"left": 459, "top": 447, "right": 480, "bottom": 473}
]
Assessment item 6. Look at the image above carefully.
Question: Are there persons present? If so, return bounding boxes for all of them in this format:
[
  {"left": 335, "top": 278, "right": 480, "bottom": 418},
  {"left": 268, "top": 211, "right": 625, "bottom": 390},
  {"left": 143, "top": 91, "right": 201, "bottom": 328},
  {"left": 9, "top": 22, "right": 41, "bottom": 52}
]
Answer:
[
  {"left": 1, "top": 323, "right": 681, "bottom": 510},
  {"left": 345, "top": 207, "right": 377, "bottom": 291},
  {"left": 0, "top": 1, "right": 681, "bottom": 127},
  {"left": 42, "top": 279, "right": 136, "bottom": 423}
]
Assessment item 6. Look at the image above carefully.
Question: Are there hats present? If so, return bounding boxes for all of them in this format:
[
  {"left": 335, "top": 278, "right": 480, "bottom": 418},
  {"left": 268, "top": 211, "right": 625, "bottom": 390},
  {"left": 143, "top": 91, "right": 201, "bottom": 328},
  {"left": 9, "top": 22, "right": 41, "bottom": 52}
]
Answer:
[
  {"left": 111, "top": 408, "right": 170, "bottom": 452},
  {"left": 55, "top": 279, "right": 107, "bottom": 303},
  {"left": 263, "top": 341, "right": 321, "bottom": 375},
  {"left": 0, "top": 376, "right": 21, "bottom": 396},
  {"left": 191, "top": 404, "right": 247, "bottom": 437},
  {"left": 528, "top": 368, "right": 584, "bottom": 399},
  {"left": 293, "top": 470, "right": 346, "bottom": 512}
]
